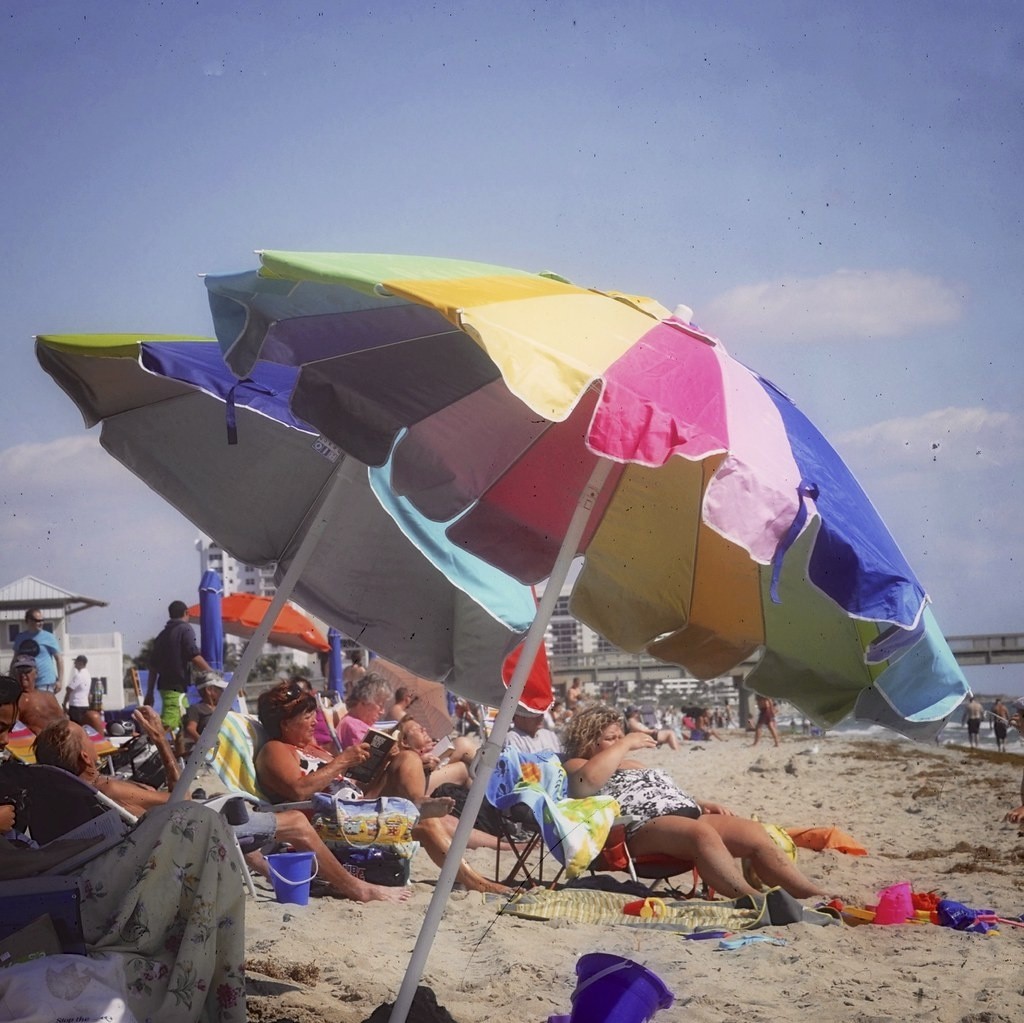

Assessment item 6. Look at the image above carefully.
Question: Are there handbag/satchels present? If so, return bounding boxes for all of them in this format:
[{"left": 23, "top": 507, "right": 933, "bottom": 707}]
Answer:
[{"left": 310, "top": 786, "right": 419, "bottom": 855}]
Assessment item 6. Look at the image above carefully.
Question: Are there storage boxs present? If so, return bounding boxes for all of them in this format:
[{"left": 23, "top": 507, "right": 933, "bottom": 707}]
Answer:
[{"left": 0, "top": 877, "right": 88, "bottom": 968}]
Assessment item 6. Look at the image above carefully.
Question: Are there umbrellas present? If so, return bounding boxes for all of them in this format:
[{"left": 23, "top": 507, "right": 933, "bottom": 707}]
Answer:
[
  {"left": 329, "top": 628, "right": 342, "bottom": 700},
  {"left": 30, "top": 335, "right": 555, "bottom": 795},
  {"left": 198, "top": 245, "right": 974, "bottom": 1023},
  {"left": 197, "top": 567, "right": 222, "bottom": 671},
  {"left": 187, "top": 592, "right": 330, "bottom": 654}
]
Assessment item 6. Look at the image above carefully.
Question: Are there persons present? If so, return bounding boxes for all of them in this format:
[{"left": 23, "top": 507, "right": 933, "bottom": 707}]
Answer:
[
  {"left": 0, "top": 601, "right": 845, "bottom": 1023},
  {"left": 1002, "top": 696, "right": 1024, "bottom": 823},
  {"left": 962, "top": 697, "right": 983, "bottom": 749},
  {"left": 989, "top": 699, "right": 1010, "bottom": 751}
]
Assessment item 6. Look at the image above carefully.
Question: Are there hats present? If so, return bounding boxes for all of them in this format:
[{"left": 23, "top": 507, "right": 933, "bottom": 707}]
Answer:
[
  {"left": 73, "top": 655, "right": 87, "bottom": 664},
  {"left": 197, "top": 678, "right": 229, "bottom": 689},
  {"left": 12, "top": 654, "right": 38, "bottom": 668}
]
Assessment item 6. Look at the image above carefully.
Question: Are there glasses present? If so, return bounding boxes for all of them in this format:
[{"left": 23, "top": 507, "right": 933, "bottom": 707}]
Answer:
[
  {"left": 283, "top": 685, "right": 301, "bottom": 704},
  {"left": 29, "top": 618, "right": 44, "bottom": 622}
]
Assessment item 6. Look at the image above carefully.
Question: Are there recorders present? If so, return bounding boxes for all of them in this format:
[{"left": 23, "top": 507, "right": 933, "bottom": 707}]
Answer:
[{"left": 106, "top": 719, "right": 137, "bottom": 736}]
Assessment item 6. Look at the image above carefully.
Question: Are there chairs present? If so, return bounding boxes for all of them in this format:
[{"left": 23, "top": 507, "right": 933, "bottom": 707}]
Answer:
[
  {"left": 17, "top": 763, "right": 257, "bottom": 898},
  {"left": 492, "top": 748, "right": 722, "bottom": 902},
  {"left": 202, "top": 712, "right": 419, "bottom": 863},
  {"left": 640, "top": 708, "right": 659, "bottom": 729},
  {"left": 621, "top": 709, "right": 630, "bottom": 736}
]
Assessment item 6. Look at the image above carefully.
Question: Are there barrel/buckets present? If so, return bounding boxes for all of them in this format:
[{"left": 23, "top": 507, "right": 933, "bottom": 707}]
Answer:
[
  {"left": 567, "top": 953, "right": 674, "bottom": 1023},
  {"left": 263, "top": 851, "right": 318, "bottom": 905}
]
